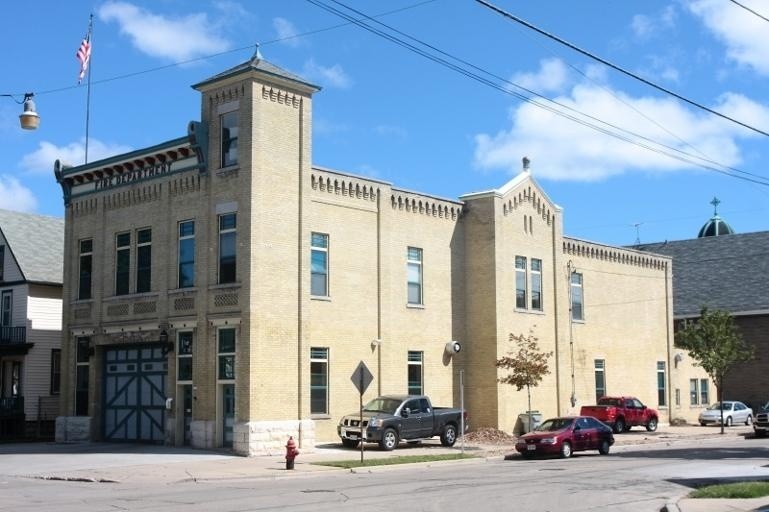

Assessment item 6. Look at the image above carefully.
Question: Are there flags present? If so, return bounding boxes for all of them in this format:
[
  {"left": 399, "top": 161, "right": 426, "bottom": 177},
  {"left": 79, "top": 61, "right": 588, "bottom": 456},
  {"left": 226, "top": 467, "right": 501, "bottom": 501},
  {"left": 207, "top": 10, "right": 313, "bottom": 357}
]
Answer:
[{"left": 75, "top": 17, "right": 92, "bottom": 86}]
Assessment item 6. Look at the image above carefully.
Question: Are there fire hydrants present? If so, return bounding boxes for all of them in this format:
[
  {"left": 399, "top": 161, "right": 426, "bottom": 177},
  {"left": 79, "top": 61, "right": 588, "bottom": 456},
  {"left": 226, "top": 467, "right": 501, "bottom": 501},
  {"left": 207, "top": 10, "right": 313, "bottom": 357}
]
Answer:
[{"left": 285, "top": 436, "right": 299, "bottom": 469}]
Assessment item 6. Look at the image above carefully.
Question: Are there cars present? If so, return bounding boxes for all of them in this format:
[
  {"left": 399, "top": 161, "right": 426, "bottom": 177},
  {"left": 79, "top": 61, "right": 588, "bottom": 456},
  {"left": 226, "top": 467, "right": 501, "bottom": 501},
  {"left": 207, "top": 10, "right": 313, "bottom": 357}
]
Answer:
[
  {"left": 699, "top": 401, "right": 753, "bottom": 426},
  {"left": 753, "top": 402, "right": 769, "bottom": 435},
  {"left": 516, "top": 416, "right": 615, "bottom": 458}
]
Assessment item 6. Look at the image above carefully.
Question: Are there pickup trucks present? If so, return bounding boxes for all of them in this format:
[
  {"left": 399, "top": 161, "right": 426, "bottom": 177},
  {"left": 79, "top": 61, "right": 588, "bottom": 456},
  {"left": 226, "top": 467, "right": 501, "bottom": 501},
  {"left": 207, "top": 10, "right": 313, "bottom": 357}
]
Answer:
[
  {"left": 580, "top": 395, "right": 659, "bottom": 433},
  {"left": 337, "top": 395, "right": 469, "bottom": 451}
]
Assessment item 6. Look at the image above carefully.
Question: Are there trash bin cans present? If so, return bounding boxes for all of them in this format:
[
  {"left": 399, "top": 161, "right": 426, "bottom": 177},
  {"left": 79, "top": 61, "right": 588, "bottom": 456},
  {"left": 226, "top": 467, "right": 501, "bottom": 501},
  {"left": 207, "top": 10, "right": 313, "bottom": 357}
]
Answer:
[{"left": 518, "top": 413, "right": 542, "bottom": 434}]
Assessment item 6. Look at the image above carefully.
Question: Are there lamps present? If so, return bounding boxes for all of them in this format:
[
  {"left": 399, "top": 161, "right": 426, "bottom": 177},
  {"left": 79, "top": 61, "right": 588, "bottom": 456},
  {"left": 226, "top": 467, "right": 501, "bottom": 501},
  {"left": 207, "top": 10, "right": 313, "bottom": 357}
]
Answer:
[{"left": 159, "top": 329, "right": 174, "bottom": 356}]
